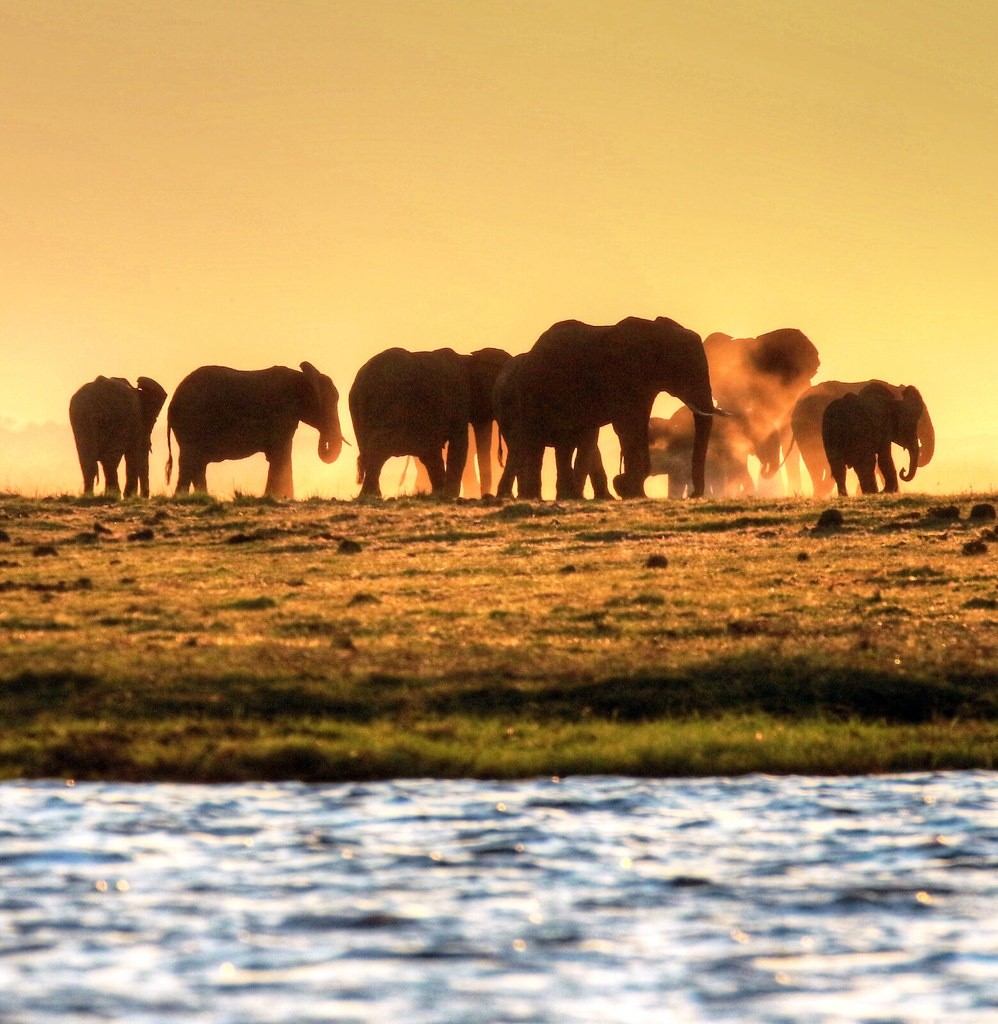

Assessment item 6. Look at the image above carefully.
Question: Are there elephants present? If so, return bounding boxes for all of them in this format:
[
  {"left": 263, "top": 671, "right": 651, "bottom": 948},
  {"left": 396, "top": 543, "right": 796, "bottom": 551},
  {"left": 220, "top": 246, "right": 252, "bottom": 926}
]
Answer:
[
  {"left": 347, "top": 316, "right": 936, "bottom": 502},
  {"left": 69, "top": 375, "right": 168, "bottom": 500},
  {"left": 164, "top": 360, "right": 352, "bottom": 503}
]
